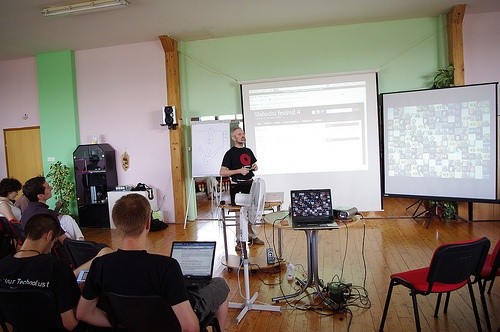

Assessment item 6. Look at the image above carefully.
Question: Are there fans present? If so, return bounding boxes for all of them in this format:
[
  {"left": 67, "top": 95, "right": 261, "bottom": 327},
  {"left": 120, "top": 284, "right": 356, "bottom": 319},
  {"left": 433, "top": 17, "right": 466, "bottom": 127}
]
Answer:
[{"left": 228, "top": 177, "right": 282, "bottom": 324}]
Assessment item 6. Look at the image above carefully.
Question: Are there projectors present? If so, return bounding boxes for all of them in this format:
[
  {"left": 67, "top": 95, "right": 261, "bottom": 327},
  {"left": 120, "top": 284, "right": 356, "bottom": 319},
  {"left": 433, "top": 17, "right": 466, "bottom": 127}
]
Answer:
[{"left": 332, "top": 206, "right": 358, "bottom": 220}]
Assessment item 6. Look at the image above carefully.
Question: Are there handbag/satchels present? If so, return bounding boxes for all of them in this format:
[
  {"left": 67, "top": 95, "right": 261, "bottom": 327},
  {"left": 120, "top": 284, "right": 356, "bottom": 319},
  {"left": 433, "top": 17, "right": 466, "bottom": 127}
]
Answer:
[
  {"left": 152, "top": 208, "right": 164, "bottom": 222},
  {"left": 149, "top": 214, "right": 168, "bottom": 232},
  {"left": 130, "top": 183, "right": 146, "bottom": 191}
]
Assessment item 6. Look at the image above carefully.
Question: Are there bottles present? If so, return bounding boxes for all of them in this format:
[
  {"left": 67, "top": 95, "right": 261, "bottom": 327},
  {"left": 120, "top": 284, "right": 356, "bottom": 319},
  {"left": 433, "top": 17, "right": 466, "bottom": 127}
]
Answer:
[{"left": 115, "top": 185, "right": 133, "bottom": 191}]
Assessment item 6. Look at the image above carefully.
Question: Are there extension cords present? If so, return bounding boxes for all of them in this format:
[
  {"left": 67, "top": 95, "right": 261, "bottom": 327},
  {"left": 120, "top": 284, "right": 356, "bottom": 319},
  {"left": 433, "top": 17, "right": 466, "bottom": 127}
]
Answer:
[{"left": 267, "top": 248, "right": 275, "bottom": 264}]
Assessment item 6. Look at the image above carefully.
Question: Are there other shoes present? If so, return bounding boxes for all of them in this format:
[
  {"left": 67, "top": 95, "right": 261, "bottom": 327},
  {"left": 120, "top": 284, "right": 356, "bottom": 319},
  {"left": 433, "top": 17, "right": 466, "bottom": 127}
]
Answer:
[
  {"left": 248, "top": 237, "right": 263, "bottom": 245},
  {"left": 235, "top": 241, "right": 245, "bottom": 250}
]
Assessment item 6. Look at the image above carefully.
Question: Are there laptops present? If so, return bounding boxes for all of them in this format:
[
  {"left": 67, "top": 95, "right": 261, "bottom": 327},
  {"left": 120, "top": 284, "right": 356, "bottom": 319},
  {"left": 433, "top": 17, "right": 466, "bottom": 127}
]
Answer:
[
  {"left": 77, "top": 269, "right": 90, "bottom": 285},
  {"left": 171, "top": 240, "right": 216, "bottom": 288},
  {"left": 291, "top": 188, "right": 340, "bottom": 230}
]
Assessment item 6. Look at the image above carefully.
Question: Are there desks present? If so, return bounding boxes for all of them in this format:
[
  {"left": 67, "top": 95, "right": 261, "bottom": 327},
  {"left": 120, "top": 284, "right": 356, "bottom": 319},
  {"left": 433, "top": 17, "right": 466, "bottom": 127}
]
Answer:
[
  {"left": 107, "top": 187, "right": 161, "bottom": 230},
  {"left": 264, "top": 211, "right": 361, "bottom": 310},
  {"left": 194, "top": 175, "right": 229, "bottom": 199}
]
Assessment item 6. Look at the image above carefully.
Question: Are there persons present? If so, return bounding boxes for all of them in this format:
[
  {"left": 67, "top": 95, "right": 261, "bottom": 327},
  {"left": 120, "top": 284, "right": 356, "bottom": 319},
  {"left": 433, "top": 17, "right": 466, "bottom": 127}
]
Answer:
[
  {"left": 220, "top": 127, "right": 264, "bottom": 251},
  {"left": 0, "top": 177, "right": 89, "bottom": 332},
  {"left": 76, "top": 194, "right": 230, "bottom": 332}
]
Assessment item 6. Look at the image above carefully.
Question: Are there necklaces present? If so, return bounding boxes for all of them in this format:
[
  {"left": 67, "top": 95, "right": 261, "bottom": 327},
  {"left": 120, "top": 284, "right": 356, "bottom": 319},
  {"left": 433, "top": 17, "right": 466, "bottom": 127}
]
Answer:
[{"left": 17, "top": 248, "right": 41, "bottom": 255}]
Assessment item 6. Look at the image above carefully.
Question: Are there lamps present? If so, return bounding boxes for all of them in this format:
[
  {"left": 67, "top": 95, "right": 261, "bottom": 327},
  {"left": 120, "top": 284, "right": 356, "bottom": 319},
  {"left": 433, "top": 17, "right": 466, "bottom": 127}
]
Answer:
[{"left": 40, "top": 0, "right": 132, "bottom": 17}]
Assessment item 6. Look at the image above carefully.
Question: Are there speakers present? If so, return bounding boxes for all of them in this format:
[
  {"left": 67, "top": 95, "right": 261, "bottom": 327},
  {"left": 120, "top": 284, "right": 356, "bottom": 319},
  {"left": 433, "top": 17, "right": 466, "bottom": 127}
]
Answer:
[{"left": 163, "top": 106, "right": 177, "bottom": 125}]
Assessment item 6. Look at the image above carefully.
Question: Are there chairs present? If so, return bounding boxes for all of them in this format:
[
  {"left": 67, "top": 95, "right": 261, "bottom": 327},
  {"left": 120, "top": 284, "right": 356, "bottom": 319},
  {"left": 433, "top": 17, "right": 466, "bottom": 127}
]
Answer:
[
  {"left": 379, "top": 236, "right": 500, "bottom": 332},
  {"left": 0, "top": 288, "right": 69, "bottom": 332},
  {"left": 104, "top": 292, "right": 222, "bottom": 332}
]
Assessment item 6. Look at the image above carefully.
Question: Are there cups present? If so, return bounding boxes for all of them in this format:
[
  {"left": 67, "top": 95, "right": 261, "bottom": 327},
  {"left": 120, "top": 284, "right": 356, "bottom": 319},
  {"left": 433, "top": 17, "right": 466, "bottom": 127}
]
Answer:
[{"left": 91, "top": 135, "right": 98, "bottom": 144}]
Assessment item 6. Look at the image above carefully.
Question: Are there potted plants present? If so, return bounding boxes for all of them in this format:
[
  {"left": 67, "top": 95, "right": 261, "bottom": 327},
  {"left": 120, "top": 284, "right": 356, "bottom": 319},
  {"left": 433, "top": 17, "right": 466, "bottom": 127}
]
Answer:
[
  {"left": 45, "top": 161, "right": 77, "bottom": 219},
  {"left": 426, "top": 66, "right": 457, "bottom": 222}
]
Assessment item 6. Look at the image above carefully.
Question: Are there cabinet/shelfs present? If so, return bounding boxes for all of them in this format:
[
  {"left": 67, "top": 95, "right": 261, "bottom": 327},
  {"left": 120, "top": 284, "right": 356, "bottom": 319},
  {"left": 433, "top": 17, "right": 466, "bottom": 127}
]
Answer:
[
  {"left": 218, "top": 200, "right": 285, "bottom": 273},
  {"left": 73, "top": 143, "right": 118, "bottom": 228}
]
